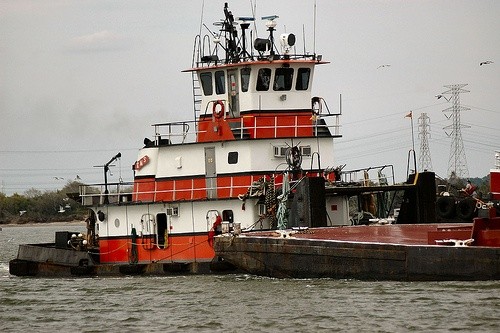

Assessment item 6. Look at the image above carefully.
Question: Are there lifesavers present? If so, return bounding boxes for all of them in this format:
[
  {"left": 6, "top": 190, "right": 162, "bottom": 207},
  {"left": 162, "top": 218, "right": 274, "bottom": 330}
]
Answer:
[
  {"left": 436, "top": 197, "right": 455, "bottom": 219},
  {"left": 213, "top": 100, "right": 225, "bottom": 119},
  {"left": 456, "top": 199, "right": 477, "bottom": 219},
  {"left": 311, "top": 97, "right": 323, "bottom": 115}
]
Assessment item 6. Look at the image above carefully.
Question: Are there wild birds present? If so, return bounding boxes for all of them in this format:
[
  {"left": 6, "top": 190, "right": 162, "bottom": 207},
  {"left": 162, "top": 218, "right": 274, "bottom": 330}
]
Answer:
[
  {"left": 377, "top": 64, "right": 391, "bottom": 70},
  {"left": 435, "top": 95, "right": 445, "bottom": 99},
  {"left": 19, "top": 210, "right": 27, "bottom": 216},
  {"left": 479, "top": 59, "right": 494, "bottom": 67},
  {"left": 212, "top": 32, "right": 219, "bottom": 37},
  {"left": 53, "top": 174, "right": 82, "bottom": 182},
  {"left": 58, "top": 197, "right": 71, "bottom": 212}
]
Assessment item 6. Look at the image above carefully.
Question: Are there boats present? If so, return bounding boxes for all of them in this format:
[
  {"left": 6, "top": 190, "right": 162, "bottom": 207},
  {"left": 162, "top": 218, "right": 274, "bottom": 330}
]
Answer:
[
  {"left": 8, "top": 0, "right": 418, "bottom": 277},
  {"left": 399, "top": 151, "right": 500, "bottom": 221},
  {"left": 0, "top": 226, "right": 2, "bottom": 231},
  {"left": 211, "top": 218, "right": 500, "bottom": 280}
]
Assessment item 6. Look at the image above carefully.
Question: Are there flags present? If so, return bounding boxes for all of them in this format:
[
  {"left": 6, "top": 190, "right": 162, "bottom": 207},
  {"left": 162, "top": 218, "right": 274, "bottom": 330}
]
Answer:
[{"left": 404, "top": 111, "right": 412, "bottom": 117}]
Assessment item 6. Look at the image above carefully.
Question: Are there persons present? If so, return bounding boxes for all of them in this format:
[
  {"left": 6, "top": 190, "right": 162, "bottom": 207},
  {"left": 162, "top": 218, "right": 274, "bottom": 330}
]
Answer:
[
  {"left": 464, "top": 180, "right": 474, "bottom": 199},
  {"left": 67, "top": 233, "right": 92, "bottom": 253}
]
